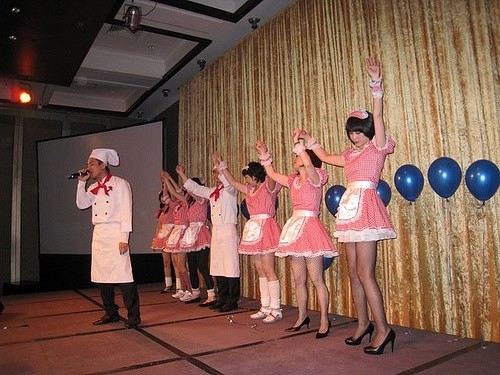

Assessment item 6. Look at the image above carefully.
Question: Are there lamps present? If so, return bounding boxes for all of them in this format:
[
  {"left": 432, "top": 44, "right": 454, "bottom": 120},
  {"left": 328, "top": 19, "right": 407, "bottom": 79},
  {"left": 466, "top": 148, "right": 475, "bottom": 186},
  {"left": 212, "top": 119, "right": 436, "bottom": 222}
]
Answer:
[
  {"left": 248, "top": 17, "right": 261, "bottom": 30},
  {"left": 137, "top": 111, "right": 143, "bottom": 118},
  {"left": 196, "top": 59, "right": 207, "bottom": 70},
  {"left": 162, "top": 89, "right": 171, "bottom": 97},
  {"left": 123, "top": 0, "right": 144, "bottom": 35}
]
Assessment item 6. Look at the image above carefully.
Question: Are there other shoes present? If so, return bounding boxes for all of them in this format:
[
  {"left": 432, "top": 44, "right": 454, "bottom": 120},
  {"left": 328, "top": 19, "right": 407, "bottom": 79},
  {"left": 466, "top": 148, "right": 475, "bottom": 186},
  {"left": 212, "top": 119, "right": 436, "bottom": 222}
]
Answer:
[
  {"left": 179, "top": 290, "right": 192, "bottom": 301},
  {"left": 160, "top": 284, "right": 174, "bottom": 295},
  {"left": 172, "top": 290, "right": 184, "bottom": 299}
]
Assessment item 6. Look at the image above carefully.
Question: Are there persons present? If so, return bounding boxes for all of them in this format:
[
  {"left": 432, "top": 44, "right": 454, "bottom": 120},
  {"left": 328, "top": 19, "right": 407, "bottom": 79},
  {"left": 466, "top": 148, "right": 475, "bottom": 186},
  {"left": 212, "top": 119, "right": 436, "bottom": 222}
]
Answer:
[
  {"left": 294, "top": 56, "right": 397, "bottom": 354},
  {"left": 214, "top": 135, "right": 284, "bottom": 324},
  {"left": 161, "top": 163, "right": 217, "bottom": 307},
  {"left": 75, "top": 148, "right": 141, "bottom": 327},
  {"left": 175, "top": 151, "right": 241, "bottom": 312},
  {"left": 151, "top": 164, "right": 185, "bottom": 301},
  {"left": 255, "top": 129, "right": 340, "bottom": 338}
]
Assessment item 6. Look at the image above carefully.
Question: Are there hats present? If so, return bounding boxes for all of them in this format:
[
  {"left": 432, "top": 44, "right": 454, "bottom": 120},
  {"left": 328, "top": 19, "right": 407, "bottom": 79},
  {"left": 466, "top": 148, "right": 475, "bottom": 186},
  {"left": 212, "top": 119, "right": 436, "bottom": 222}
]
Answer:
[{"left": 90, "top": 148, "right": 120, "bottom": 167}]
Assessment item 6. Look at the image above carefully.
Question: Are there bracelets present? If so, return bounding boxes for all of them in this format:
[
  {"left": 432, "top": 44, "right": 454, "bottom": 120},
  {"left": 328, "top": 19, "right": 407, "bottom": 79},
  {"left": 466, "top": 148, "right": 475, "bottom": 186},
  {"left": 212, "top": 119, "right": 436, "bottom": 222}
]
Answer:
[
  {"left": 293, "top": 141, "right": 306, "bottom": 155},
  {"left": 370, "top": 74, "right": 383, "bottom": 98},
  {"left": 219, "top": 161, "right": 228, "bottom": 170},
  {"left": 305, "top": 138, "right": 320, "bottom": 150},
  {"left": 258, "top": 154, "right": 272, "bottom": 165}
]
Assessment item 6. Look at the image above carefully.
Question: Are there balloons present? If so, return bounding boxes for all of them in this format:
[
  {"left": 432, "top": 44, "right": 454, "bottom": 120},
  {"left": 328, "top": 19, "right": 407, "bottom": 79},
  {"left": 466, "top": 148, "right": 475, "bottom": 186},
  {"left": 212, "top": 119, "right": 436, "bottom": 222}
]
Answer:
[
  {"left": 376, "top": 180, "right": 392, "bottom": 207},
  {"left": 241, "top": 199, "right": 250, "bottom": 219},
  {"left": 465, "top": 159, "right": 500, "bottom": 202},
  {"left": 323, "top": 257, "right": 334, "bottom": 270},
  {"left": 394, "top": 164, "right": 424, "bottom": 201},
  {"left": 325, "top": 185, "right": 347, "bottom": 218},
  {"left": 207, "top": 204, "right": 211, "bottom": 221},
  {"left": 428, "top": 157, "right": 462, "bottom": 198}
]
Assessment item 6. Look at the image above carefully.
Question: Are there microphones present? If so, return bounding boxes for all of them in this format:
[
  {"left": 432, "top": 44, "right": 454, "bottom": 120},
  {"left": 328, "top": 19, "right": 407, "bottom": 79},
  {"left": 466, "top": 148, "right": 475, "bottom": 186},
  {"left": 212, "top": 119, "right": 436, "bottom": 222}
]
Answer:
[{"left": 68, "top": 170, "right": 92, "bottom": 179}]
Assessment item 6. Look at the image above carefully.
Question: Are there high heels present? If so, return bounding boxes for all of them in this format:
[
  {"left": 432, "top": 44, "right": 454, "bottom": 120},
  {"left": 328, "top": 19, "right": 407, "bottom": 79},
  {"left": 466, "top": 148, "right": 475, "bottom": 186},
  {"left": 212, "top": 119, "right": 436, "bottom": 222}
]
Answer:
[
  {"left": 363, "top": 328, "right": 396, "bottom": 355},
  {"left": 315, "top": 317, "right": 331, "bottom": 339},
  {"left": 285, "top": 315, "right": 311, "bottom": 332},
  {"left": 344, "top": 321, "right": 374, "bottom": 346}
]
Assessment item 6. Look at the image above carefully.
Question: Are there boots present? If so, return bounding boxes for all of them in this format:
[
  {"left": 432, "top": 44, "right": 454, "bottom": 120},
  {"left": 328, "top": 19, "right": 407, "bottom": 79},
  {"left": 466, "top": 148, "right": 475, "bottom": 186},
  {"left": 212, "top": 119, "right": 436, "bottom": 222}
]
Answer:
[
  {"left": 198, "top": 289, "right": 217, "bottom": 307},
  {"left": 183, "top": 287, "right": 202, "bottom": 304}
]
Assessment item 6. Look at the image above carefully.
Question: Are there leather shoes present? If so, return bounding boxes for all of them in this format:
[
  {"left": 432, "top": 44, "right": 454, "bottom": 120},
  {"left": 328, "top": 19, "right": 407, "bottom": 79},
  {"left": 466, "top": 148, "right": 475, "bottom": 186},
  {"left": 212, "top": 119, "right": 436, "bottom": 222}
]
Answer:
[
  {"left": 127, "top": 316, "right": 141, "bottom": 328},
  {"left": 92, "top": 313, "right": 120, "bottom": 325},
  {"left": 209, "top": 302, "right": 225, "bottom": 309},
  {"left": 219, "top": 303, "right": 238, "bottom": 312}
]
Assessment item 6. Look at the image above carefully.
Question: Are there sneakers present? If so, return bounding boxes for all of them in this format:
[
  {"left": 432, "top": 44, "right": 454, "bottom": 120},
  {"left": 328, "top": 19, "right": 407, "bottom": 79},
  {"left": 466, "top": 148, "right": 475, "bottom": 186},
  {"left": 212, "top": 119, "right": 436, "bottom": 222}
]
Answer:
[
  {"left": 263, "top": 308, "right": 284, "bottom": 323},
  {"left": 250, "top": 307, "right": 270, "bottom": 319}
]
